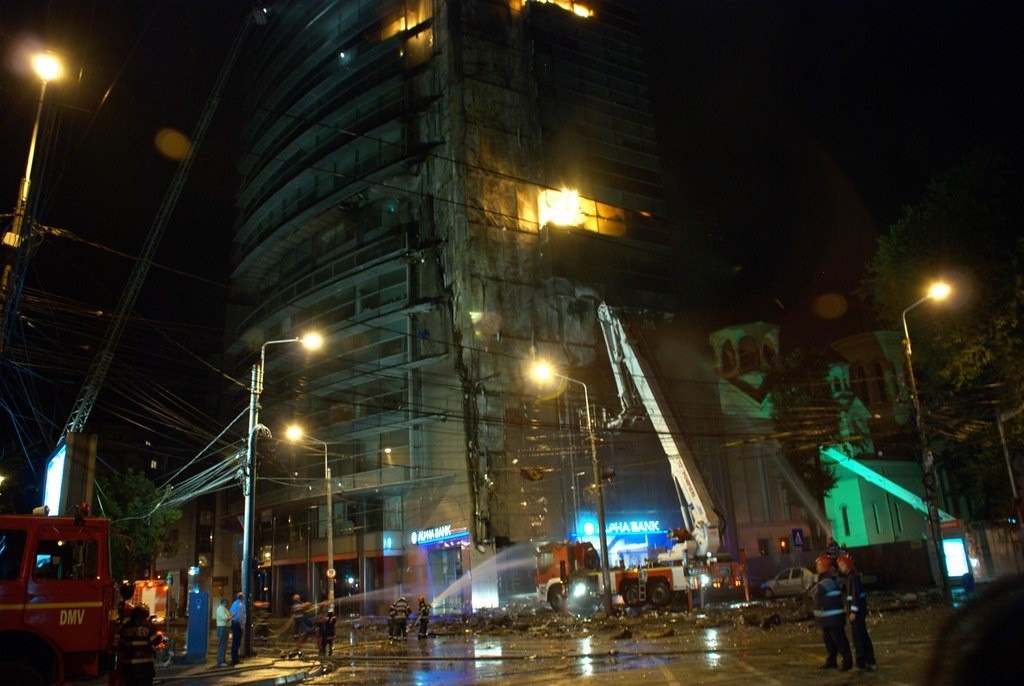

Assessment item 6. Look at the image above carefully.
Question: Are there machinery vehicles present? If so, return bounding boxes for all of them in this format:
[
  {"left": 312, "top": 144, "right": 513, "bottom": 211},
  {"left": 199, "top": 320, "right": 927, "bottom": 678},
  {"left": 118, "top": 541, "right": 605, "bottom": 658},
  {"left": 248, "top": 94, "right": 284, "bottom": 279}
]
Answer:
[{"left": 535, "top": 301, "right": 744, "bottom": 617}]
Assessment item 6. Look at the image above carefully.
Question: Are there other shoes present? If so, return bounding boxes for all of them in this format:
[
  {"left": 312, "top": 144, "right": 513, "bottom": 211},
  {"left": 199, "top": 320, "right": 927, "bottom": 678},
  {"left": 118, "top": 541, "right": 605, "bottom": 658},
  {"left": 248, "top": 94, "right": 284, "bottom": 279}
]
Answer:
[
  {"left": 820, "top": 662, "right": 837, "bottom": 669},
  {"left": 232, "top": 660, "right": 243, "bottom": 664},
  {"left": 839, "top": 665, "right": 852, "bottom": 673},
  {"left": 217, "top": 662, "right": 228, "bottom": 667},
  {"left": 867, "top": 663, "right": 876, "bottom": 673}
]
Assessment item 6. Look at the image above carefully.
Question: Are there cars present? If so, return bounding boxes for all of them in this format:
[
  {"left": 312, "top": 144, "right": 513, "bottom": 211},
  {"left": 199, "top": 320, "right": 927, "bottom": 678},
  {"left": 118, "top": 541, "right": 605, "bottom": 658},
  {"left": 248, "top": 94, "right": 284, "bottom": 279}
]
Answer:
[{"left": 758, "top": 566, "right": 818, "bottom": 600}]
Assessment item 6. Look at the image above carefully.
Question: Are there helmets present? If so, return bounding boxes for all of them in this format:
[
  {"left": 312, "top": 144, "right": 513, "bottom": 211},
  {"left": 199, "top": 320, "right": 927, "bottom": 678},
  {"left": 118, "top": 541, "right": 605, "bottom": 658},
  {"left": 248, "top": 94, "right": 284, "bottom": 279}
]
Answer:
[
  {"left": 293, "top": 594, "right": 300, "bottom": 601},
  {"left": 131, "top": 606, "right": 149, "bottom": 620},
  {"left": 816, "top": 555, "right": 832, "bottom": 568},
  {"left": 836, "top": 555, "right": 852, "bottom": 569},
  {"left": 418, "top": 595, "right": 424, "bottom": 602}
]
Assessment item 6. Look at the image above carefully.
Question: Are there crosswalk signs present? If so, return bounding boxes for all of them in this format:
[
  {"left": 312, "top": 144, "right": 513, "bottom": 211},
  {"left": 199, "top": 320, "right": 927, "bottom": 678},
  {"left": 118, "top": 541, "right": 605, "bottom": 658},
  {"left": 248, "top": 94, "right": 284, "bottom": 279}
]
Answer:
[{"left": 793, "top": 529, "right": 804, "bottom": 546}]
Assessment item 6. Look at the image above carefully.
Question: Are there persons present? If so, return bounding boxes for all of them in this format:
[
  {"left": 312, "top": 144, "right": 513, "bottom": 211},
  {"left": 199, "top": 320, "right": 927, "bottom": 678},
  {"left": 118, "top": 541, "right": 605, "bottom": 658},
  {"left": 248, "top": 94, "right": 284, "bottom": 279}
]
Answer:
[
  {"left": 229, "top": 592, "right": 246, "bottom": 664},
  {"left": 314, "top": 613, "right": 337, "bottom": 656},
  {"left": 388, "top": 597, "right": 411, "bottom": 641},
  {"left": 837, "top": 555, "right": 877, "bottom": 672},
  {"left": 216, "top": 598, "right": 237, "bottom": 666},
  {"left": 925, "top": 574, "right": 1024, "bottom": 686},
  {"left": 107, "top": 603, "right": 163, "bottom": 686},
  {"left": 418, "top": 595, "right": 431, "bottom": 639},
  {"left": 814, "top": 555, "right": 853, "bottom": 671},
  {"left": 293, "top": 595, "right": 307, "bottom": 641}
]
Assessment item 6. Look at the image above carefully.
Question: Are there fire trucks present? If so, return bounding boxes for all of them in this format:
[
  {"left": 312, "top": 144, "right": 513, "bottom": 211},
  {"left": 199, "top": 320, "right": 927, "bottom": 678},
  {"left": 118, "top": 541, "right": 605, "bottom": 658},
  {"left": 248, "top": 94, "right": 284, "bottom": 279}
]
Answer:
[
  {"left": 130, "top": 579, "right": 177, "bottom": 629},
  {"left": 0, "top": 502, "right": 134, "bottom": 686}
]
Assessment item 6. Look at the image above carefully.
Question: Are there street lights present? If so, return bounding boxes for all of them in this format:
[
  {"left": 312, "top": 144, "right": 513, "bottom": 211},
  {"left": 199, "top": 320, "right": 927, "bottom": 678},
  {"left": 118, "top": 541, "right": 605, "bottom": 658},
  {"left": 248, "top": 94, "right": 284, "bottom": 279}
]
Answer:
[
  {"left": 285, "top": 427, "right": 335, "bottom": 615},
  {"left": 0, "top": 49, "right": 64, "bottom": 345},
  {"left": 902, "top": 285, "right": 950, "bottom": 599},
  {"left": 241, "top": 334, "right": 323, "bottom": 657},
  {"left": 531, "top": 364, "right": 612, "bottom": 620}
]
